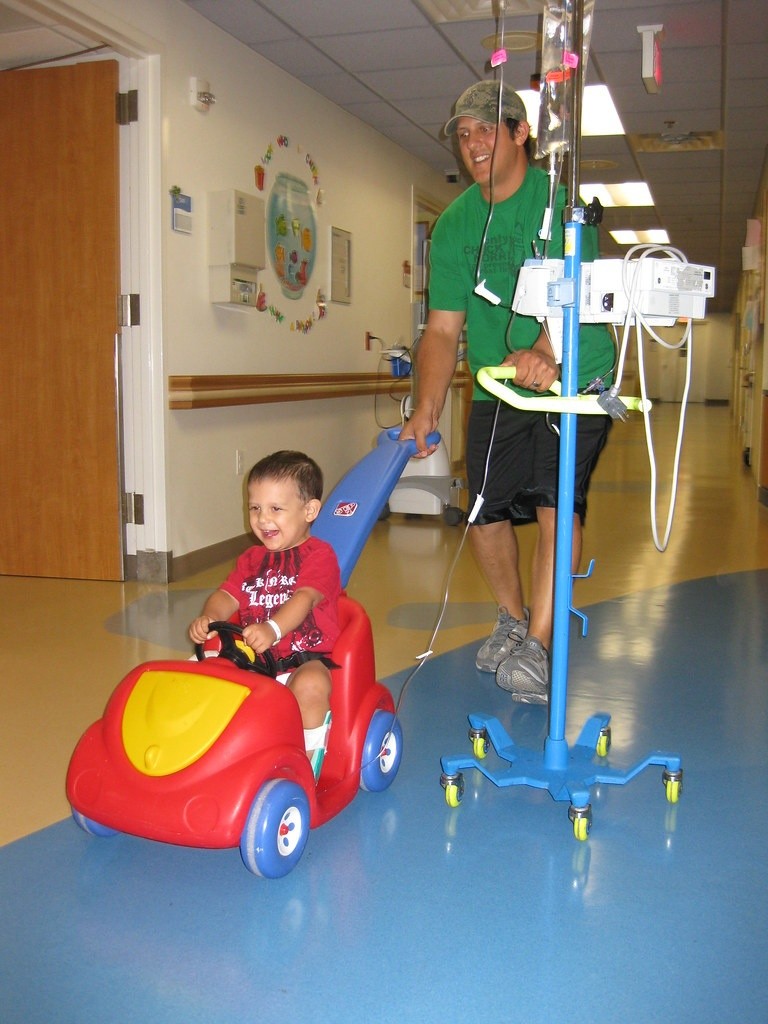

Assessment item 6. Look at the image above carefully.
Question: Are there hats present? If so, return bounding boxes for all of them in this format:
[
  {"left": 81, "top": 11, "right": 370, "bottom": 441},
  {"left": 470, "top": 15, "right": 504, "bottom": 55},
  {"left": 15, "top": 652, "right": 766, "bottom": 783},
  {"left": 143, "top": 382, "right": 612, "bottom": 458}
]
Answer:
[{"left": 444, "top": 80, "right": 527, "bottom": 136}]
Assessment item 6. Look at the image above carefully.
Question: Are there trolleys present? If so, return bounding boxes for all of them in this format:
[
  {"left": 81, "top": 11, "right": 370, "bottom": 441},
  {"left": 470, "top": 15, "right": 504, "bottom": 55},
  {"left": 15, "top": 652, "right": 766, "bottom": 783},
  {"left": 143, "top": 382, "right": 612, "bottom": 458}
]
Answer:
[{"left": 64, "top": 426, "right": 445, "bottom": 886}]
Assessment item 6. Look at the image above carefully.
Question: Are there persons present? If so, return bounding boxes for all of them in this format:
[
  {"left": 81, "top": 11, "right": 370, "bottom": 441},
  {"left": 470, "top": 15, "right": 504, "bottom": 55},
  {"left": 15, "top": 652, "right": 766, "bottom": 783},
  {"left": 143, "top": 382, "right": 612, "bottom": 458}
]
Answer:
[
  {"left": 399, "top": 81, "right": 616, "bottom": 706},
  {"left": 190, "top": 450, "right": 341, "bottom": 760}
]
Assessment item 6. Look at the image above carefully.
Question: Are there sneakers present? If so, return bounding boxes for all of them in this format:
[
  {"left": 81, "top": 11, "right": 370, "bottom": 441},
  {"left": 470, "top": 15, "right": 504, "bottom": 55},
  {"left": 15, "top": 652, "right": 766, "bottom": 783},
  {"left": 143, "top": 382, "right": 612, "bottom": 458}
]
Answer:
[
  {"left": 476, "top": 606, "right": 530, "bottom": 673},
  {"left": 496, "top": 631, "right": 551, "bottom": 705}
]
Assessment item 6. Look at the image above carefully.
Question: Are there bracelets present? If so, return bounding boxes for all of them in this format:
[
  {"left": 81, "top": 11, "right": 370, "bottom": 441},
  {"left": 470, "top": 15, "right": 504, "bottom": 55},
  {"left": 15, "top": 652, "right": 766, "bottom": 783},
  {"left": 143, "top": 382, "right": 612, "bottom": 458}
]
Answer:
[{"left": 266, "top": 620, "right": 282, "bottom": 647}]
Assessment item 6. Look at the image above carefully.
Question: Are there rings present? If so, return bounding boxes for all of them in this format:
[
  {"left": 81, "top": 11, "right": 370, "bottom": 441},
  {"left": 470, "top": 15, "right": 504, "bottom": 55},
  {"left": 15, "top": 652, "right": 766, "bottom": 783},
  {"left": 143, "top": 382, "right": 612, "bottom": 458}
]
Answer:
[{"left": 532, "top": 382, "right": 541, "bottom": 386}]
